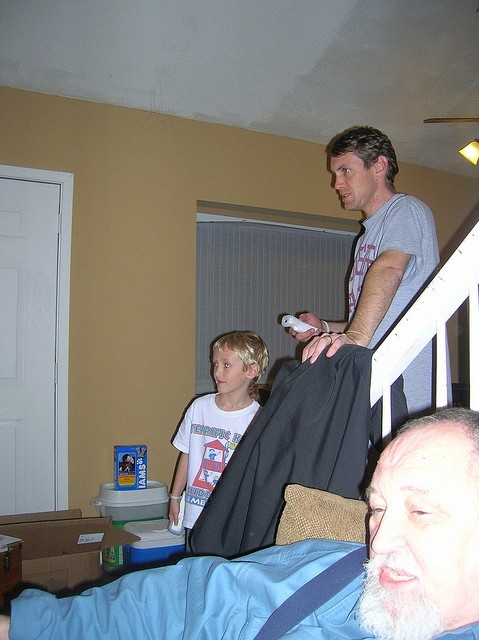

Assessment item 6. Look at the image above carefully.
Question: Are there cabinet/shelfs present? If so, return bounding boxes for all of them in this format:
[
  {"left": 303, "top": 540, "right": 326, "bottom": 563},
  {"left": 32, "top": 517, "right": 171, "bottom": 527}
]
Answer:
[{"left": 0, "top": 534, "right": 22, "bottom": 614}]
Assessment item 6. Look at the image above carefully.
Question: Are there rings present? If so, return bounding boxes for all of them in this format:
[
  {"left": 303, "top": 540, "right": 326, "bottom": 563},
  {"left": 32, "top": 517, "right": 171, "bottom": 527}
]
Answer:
[{"left": 324, "top": 335, "right": 333, "bottom": 346}]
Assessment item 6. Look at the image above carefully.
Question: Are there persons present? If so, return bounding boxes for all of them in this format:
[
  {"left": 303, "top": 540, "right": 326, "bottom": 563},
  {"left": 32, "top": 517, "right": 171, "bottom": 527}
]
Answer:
[
  {"left": 0, "top": 407, "right": 477, "bottom": 639},
  {"left": 168, "top": 331, "right": 270, "bottom": 559},
  {"left": 122, "top": 454, "right": 135, "bottom": 472},
  {"left": 287, "top": 125, "right": 453, "bottom": 415}
]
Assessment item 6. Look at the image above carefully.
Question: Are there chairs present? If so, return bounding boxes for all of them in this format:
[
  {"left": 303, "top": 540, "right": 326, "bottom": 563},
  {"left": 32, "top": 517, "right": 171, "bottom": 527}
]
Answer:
[{"left": 277, "top": 485, "right": 369, "bottom": 545}]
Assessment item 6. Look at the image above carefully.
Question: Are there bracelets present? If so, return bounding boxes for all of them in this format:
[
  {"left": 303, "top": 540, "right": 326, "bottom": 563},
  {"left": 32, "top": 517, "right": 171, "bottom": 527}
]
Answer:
[
  {"left": 346, "top": 330, "right": 370, "bottom": 343},
  {"left": 167, "top": 492, "right": 183, "bottom": 500},
  {"left": 321, "top": 319, "right": 329, "bottom": 334}
]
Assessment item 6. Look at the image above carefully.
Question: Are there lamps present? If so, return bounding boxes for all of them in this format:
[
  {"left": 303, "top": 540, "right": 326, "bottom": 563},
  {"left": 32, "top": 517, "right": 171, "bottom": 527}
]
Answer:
[
  {"left": 458, "top": 139, "right": 479, "bottom": 165},
  {"left": 424, "top": 118, "right": 478, "bottom": 124}
]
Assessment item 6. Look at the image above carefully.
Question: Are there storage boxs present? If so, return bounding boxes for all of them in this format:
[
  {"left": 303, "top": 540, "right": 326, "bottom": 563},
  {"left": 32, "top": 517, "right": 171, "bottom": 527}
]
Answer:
[
  {"left": 0, "top": 509, "right": 141, "bottom": 595},
  {"left": 122, "top": 520, "right": 186, "bottom": 572}
]
